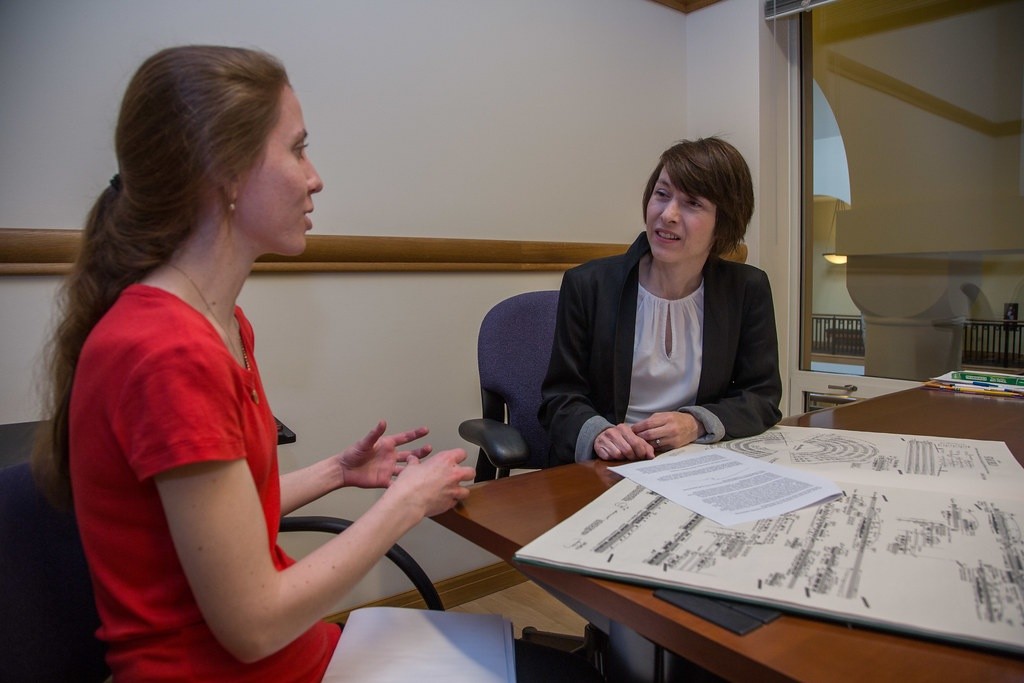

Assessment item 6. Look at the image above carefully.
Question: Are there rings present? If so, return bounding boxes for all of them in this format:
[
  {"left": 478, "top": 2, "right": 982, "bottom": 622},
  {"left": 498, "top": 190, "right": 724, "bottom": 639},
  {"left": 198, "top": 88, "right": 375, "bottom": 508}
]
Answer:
[{"left": 657, "top": 439, "right": 661, "bottom": 448}]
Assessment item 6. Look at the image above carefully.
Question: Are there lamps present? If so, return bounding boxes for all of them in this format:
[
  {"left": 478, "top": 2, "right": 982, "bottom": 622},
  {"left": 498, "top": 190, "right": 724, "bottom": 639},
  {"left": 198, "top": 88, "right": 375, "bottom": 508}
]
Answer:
[{"left": 820, "top": 197, "right": 849, "bottom": 266}]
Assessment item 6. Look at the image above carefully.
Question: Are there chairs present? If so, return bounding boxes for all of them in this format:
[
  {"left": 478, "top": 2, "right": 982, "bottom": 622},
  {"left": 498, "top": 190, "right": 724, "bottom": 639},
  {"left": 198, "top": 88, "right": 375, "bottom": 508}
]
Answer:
[
  {"left": 458, "top": 289, "right": 560, "bottom": 483},
  {"left": 0, "top": 417, "right": 443, "bottom": 683}
]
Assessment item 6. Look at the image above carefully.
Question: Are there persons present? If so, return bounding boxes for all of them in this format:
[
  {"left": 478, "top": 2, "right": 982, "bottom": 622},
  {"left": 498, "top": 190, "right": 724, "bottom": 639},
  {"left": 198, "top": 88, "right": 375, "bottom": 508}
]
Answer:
[
  {"left": 32, "top": 47, "right": 603, "bottom": 683},
  {"left": 538, "top": 137, "right": 783, "bottom": 462}
]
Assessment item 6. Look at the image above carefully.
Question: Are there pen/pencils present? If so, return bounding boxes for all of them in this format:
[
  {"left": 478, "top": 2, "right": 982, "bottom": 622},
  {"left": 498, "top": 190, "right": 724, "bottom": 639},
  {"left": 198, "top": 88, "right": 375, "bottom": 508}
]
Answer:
[{"left": 940, "top": 382, "right": 1024, "bottom": 397}]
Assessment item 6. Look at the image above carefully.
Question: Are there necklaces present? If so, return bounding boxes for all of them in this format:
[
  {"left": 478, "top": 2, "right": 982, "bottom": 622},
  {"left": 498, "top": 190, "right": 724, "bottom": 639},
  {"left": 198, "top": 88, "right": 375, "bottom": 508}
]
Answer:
[{"left": 168, "top": 264, "right": 259, "bottom": 404}]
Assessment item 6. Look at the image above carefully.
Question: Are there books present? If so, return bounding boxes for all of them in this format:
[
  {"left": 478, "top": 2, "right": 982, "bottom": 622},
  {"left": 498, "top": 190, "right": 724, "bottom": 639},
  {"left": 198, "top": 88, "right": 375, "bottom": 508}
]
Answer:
[
  {"left": 514, "top": 424, "right": 1023, "bottom": 659},
  {"left": 951, "top": 370, "right": 1024, "bottom": 385}
]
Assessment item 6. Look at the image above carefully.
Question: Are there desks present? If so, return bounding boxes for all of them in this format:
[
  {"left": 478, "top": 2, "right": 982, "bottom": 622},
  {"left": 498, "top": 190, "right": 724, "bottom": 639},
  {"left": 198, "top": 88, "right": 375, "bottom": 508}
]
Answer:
[{"left": 424, "top": 384, "right": 1023, "bottom": 683}]
